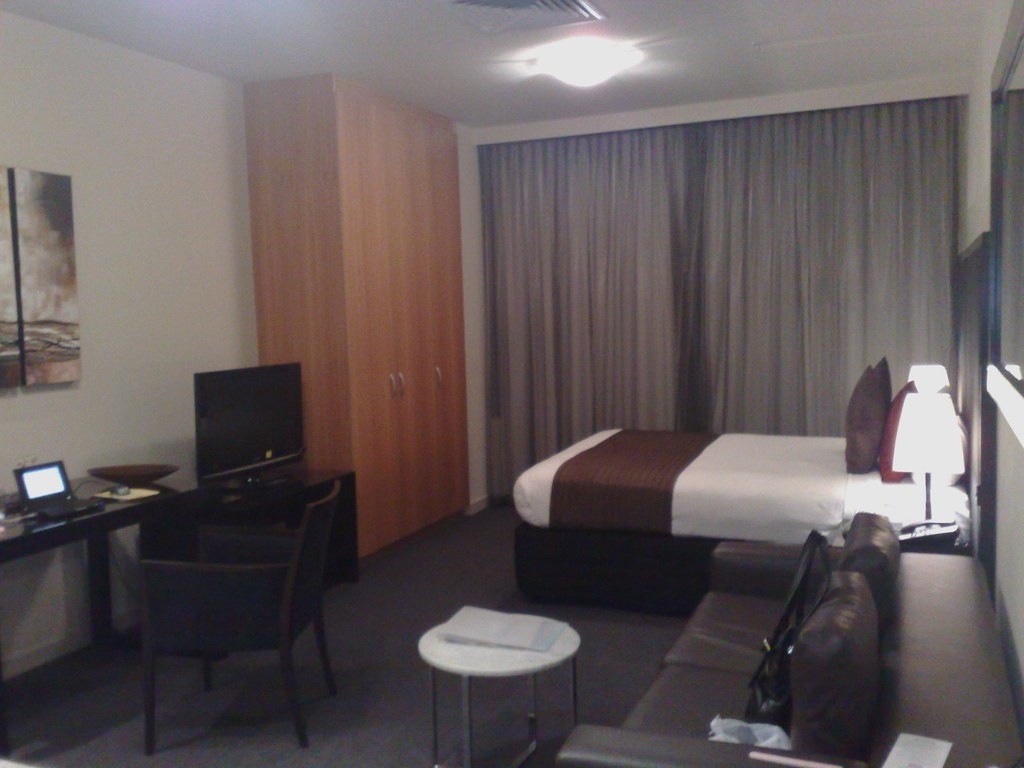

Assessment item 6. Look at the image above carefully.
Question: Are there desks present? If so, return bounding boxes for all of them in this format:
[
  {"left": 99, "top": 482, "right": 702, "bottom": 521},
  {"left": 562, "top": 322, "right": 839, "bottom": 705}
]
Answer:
[{"left": 0, "top": 476, "right": 165, "bottom": 755}]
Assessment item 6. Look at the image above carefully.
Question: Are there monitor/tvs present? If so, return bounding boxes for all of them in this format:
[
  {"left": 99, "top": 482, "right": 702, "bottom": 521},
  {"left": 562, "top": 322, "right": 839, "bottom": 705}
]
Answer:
[{"left": 193, "top": 362, "right": 305, "bottom": 482}]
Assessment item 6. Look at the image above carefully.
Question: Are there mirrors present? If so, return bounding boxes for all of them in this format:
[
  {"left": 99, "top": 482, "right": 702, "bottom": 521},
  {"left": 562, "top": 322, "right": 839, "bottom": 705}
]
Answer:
[{"left": 989, "top": 0, "right": 1024, "bottom": 400}]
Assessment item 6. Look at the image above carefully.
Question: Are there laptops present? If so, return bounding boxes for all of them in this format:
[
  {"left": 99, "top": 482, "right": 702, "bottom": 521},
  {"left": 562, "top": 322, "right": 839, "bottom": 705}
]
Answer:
[{"left": 13, "top": 460, "right": 103, "bottom": 519}]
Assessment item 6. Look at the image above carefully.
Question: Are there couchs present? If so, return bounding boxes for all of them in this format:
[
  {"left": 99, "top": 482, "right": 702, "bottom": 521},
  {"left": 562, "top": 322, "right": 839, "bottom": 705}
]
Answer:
[{"left": 555, "top": 553, "right": 1024, "bottom": 768}]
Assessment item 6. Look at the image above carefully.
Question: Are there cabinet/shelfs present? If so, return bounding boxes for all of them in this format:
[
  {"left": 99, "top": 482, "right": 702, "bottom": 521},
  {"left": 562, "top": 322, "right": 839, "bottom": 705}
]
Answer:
[
  {"left": 242, "top": 74, "right": 468, "bottom": 573},
  {"left": 140, "top": 470, "right": 360, "bottom": 658}
]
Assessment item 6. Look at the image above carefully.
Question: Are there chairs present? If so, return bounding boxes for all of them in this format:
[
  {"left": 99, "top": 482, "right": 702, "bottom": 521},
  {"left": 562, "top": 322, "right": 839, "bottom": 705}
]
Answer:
[{"left": 140, "top": 478, "right": 338, "bottom": 758}]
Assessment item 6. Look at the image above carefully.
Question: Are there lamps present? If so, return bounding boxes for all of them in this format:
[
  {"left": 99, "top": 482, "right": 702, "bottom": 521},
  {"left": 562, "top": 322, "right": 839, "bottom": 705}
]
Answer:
[
  {"left": 892, "top": 393, "right": 965, "bottom": 527},
  {"left": 908, "top": 364, "right": 950, "bottom": 394},
  {"left": 1004, "top": 365, "right": 1022, "bottom": 381}
]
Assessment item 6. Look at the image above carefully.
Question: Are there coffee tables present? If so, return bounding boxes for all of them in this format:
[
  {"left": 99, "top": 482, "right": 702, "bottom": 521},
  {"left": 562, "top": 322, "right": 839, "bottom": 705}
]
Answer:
[{"left": 418, "top": 614, "right": 580, "bottom": 767}]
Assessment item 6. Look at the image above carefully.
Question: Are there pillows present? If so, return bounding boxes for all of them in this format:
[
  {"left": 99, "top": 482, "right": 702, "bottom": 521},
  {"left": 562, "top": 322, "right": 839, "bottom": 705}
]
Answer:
[
  {"left": 878, "top": 381, "right": 918, "bottom": 484},
  {"left": 837, "top": 512, "right": 900, "bottom": 639},
  {"left": 844, "top": 356, "right": 892, "bottom": 474},
  {"left": 912, "top": 416, "right": 968, "bottom": 487},
  {"left": 789, "top": 571, "right": 879, "bottom": 757}
]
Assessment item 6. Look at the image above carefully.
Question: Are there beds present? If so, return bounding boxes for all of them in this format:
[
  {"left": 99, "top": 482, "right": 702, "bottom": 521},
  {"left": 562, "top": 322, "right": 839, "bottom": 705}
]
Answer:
[{"left": 513, "top": 425, "right": 972, "bottom": 616}]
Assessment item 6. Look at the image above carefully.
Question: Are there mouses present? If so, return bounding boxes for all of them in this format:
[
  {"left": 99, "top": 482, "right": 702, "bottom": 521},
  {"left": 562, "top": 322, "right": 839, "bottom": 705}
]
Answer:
[{"left": 109, "top": 485, "right": 130, "bottom": 495}]
